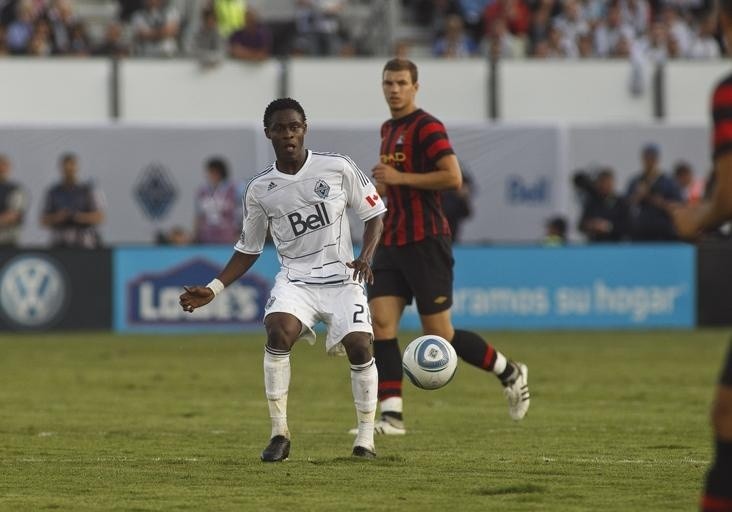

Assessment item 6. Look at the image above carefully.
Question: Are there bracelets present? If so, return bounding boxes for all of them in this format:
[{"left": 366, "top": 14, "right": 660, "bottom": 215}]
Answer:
[{"left": 205, "top": 277, "right": 226, "bottom": 297}]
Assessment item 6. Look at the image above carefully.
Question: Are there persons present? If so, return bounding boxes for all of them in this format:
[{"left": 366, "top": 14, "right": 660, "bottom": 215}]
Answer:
[
  {"left": 347, "top": 58, "right": 531, "bottom": 436},
  {"left": 193, "top": 154, "right": 249, "bottom": 243},
  {"left": 537, "top": 140, "right": 731, "bottom": 247},
  {"left": 36, "top": 152, "right": 107, "bottom": 251},
  {"left": 667, "top": 1, "right": 732, "bottom": 512},
  {"left": 178, "top": 95, "right": 389, "bottom": 461},
  {"left": 0, "top": 151, "right": 31, "bottom": 245}
]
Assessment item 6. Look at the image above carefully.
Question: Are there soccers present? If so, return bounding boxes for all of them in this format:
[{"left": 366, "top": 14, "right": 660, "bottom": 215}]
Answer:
[{"left": 402, "top": 335, "right": 457, "bottom": 390}]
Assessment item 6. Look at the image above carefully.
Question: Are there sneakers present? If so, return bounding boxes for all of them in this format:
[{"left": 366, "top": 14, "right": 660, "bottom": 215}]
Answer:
[
  {"left": 502, "top": 359, "right": 532, "bottom": 422},
  {"left": 257, "top": 434, "right": 294, "bottom": 462},
  {"left": 344, "top": 444, "right": 379, "bottom": 460},
  {"left": 348, "top": 418, "right": 409, "bottom": 437}
]
